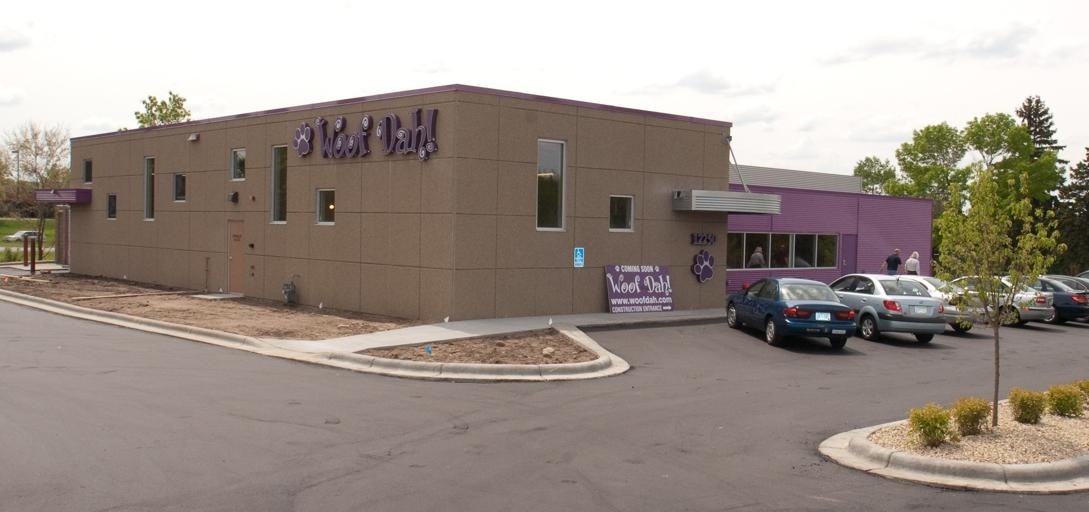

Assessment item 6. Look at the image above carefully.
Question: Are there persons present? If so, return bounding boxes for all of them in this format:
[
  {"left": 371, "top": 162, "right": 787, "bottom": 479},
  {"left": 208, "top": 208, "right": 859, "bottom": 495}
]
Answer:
[
  {"left": 747, "top": 247, "right": 766, "bottom": 269},
  {"left": 905, "top": 251, "right": 920, "bottom": 275},
  {"left": 879, "top": 249, "right": 902, "bottom": 274},
  {"left": 773, "top": 245, "right": 788, "bottom": 268}
]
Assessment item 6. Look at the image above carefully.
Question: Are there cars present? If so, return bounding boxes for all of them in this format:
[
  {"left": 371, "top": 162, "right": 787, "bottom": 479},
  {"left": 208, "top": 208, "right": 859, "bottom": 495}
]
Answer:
[
  {"left": 827, "top": 273, "right": 994, "bottom": 344},
  {"left": 726, "top": 276, "right": 857, "bottom": 350},
  {"left": 1002, "top": 270, "right": 1089, "bottom": 324},
  {"left": 938, "top": 275, "right": 1055, "bottom": 327}
]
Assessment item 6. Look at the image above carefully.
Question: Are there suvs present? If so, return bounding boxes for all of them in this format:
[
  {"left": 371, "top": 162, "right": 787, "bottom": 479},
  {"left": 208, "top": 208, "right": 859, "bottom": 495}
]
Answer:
[{"left": 4, "top": 230, "right": 46, "bottom": 243}]
